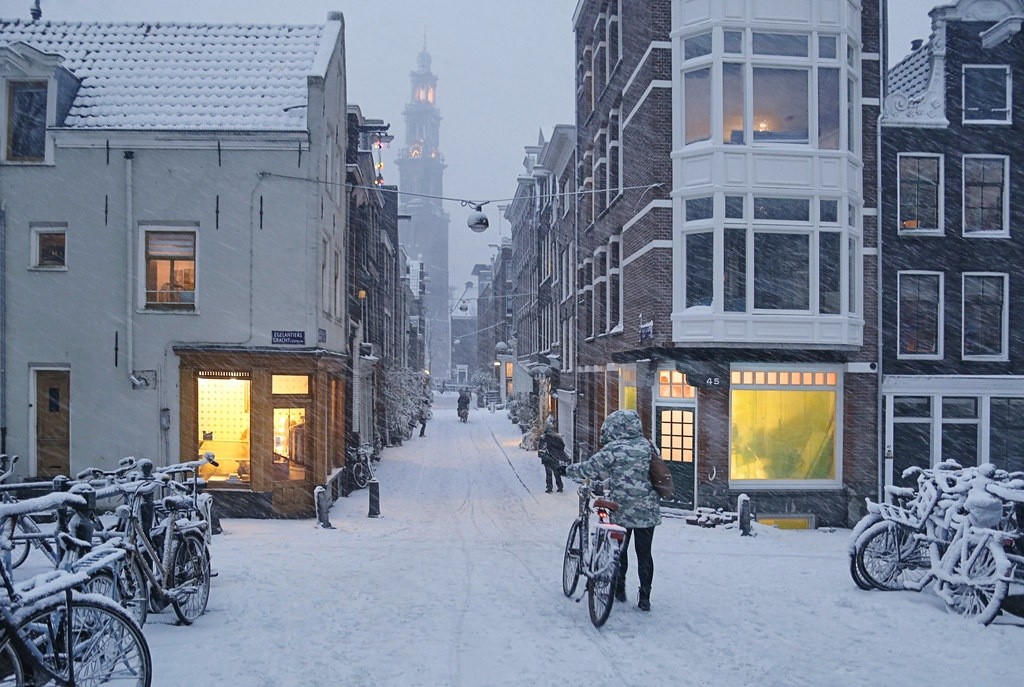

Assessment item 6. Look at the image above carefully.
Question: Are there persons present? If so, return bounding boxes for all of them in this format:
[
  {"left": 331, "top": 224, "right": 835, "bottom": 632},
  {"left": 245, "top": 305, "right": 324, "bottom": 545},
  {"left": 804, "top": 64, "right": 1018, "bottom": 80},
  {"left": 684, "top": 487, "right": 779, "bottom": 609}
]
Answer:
[
  {"left": 567, "top": 409, "right": 662, "bottom": 611},
  {"left": 419, "top": 419, "right": 426, "bottom": 437},
  {"left": 539, "top": 425, "right": 565, "bottom": 494},
  {"left": 457, "top": 387, "right": 472, "bottom": 423}
]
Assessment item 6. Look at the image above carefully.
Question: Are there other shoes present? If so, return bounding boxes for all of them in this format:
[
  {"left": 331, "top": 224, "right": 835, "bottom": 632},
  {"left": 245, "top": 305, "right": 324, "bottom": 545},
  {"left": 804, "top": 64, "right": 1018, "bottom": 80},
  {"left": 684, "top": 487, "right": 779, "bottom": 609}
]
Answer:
[
  {"left": 545, "top": 489, "right": 553, "bottom": 492},
  {"left": 557, "top": 489, "right": 563, "bottom": 493}
]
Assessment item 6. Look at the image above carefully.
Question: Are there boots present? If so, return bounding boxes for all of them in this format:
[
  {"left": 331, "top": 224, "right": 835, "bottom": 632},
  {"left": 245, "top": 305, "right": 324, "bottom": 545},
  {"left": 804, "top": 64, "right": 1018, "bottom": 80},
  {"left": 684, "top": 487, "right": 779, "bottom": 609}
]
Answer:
[
  {"left": 638, "top": 586, "right": 651, "bottom": 611},
  {"left": 615, "top": 584, "right": 627, "bottom": 602}
]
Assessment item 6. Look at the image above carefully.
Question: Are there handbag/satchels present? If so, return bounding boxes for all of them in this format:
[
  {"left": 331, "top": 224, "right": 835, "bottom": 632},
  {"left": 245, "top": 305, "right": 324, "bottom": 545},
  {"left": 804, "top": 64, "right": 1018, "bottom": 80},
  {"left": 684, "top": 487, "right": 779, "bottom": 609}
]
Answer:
[{"left": 648, "top": 437, "right": 676, "bottom": 501}]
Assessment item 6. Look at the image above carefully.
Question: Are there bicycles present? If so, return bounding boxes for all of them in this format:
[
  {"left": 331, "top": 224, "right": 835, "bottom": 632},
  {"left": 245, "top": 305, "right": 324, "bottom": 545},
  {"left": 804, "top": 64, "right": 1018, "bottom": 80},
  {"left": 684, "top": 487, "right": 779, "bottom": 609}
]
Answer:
[
  {"left": 352, "top": 443, "right": 375, "bottom": 489},
  {"left": 390, "top": 394, "right": 432, "bottom": 446},
  {"left": 460, "top": 407, "right": 469, "bottom": 423},
  {"left": 847, "top": 459, "right": 1024, "bottom": 626},
  {"left": 562, "top": 476, "right": 628, "bottom": 626},
  {"left": 0, "top": 453, "right": 220, "bottom": 686}
]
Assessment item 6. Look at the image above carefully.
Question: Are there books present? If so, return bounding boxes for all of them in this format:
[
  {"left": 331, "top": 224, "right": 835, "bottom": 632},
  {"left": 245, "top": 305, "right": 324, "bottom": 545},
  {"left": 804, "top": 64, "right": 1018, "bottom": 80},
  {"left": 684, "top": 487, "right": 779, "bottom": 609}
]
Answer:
[{"left": 226, "top": 473, "right": 242, "bottom": 483}]
